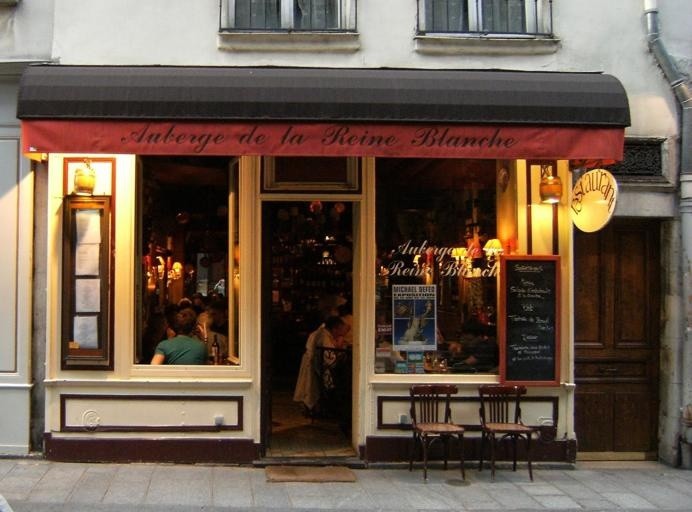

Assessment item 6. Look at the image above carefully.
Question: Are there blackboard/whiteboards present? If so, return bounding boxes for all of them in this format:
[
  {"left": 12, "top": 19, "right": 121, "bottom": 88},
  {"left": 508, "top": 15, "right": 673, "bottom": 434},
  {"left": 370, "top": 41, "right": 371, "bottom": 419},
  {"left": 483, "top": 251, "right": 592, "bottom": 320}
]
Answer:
[{"left": 499, "top": 254, "right": 562, "bottom": 387}]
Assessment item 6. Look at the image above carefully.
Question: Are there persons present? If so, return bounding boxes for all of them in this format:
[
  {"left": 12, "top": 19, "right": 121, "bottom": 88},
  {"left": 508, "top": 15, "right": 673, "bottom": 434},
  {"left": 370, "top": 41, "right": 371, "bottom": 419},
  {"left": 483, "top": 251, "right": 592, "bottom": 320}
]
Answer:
[
  {"left": 278, "top": 284, "right": 499, "bottom": 421},
  {"left": 149, "top": 289, "right": 228, "bottom": 365}
]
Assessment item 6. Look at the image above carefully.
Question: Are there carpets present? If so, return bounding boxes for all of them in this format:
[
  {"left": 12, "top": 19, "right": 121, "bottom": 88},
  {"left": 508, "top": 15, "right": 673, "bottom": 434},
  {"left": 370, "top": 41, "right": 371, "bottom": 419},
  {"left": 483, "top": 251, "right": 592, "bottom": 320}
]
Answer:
[{"left": 265, "top": 465, "right": 355, "bottom": 482}]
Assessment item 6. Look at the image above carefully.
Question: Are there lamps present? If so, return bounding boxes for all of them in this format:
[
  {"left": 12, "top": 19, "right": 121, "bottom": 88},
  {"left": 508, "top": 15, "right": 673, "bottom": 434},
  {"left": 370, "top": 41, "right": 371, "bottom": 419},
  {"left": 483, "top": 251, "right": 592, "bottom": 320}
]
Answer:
[
  {"left": 73, "top": 158, "right": 94, "bottom": 194},
  {"left": 528, "top": 176, "right": 563, "bottom": 205}
]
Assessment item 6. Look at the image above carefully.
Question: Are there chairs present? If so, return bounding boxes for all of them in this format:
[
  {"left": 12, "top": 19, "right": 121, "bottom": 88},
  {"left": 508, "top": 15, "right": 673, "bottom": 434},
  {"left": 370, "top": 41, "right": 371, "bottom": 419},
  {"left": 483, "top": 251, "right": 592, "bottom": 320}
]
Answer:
[
  {"left": 409, "top": 386, "right": 539, "bottom": 483},
  {"left": 310, "top": 347, "right": 351, "bottom": 424}
]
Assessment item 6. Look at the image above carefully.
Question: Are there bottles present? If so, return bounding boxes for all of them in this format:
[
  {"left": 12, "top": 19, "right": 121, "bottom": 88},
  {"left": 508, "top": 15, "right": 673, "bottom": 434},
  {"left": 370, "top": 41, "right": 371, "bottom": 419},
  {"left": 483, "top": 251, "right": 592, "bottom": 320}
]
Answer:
[
  {"left": 207, "top": 333, "right": 220, "bottom": 365},
  {"left": 425, "top": 350, "right": 447, "bottom": 371}
]
